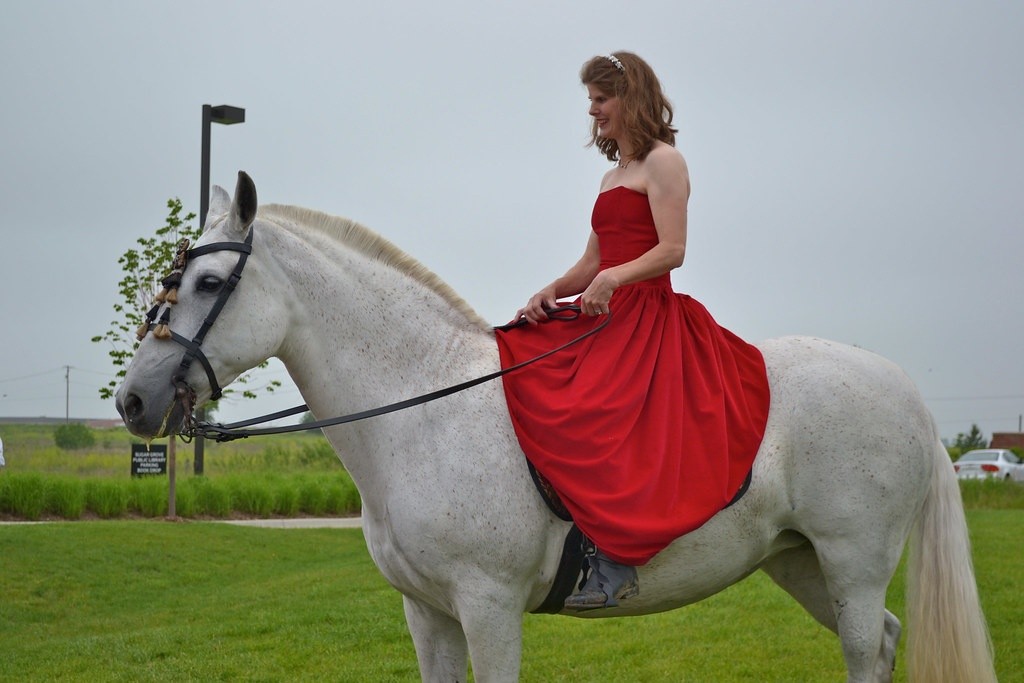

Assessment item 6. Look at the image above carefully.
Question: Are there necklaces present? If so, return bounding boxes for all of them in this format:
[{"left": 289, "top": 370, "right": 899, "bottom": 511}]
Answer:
[{"left": 617, "top": 157, "right": 636, "bottom": 170}]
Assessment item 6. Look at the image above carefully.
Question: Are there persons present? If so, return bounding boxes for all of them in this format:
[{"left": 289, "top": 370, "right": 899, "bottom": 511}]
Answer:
[{"left": 495, "top": 50, "right": 771, "bottom": 609}]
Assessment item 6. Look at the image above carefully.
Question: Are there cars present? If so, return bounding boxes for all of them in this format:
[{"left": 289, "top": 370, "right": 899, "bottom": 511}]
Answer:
[{"left": 951, "top": 449, "right": 1024, "bottom": 484}]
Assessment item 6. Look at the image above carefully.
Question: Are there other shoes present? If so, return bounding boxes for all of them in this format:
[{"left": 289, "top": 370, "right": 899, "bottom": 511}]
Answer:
[{"left": 566, "top": 556, "right": 640, "bottom": 608}]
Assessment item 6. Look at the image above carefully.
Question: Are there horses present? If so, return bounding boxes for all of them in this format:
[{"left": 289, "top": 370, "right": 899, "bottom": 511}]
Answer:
[{"left": 115, "top": 170, "right": 999, "bottom": 683}]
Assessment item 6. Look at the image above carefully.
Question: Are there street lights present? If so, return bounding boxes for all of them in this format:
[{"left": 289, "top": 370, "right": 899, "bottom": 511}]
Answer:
[{"left": 192, "top": 102, "right": 247, "bottom": 476}]
